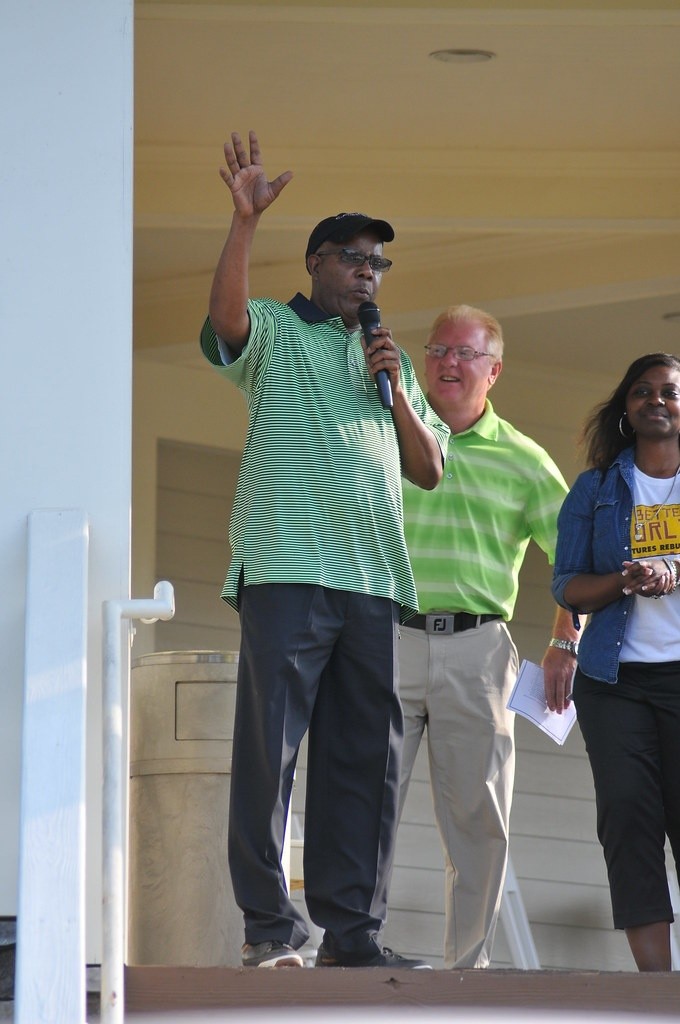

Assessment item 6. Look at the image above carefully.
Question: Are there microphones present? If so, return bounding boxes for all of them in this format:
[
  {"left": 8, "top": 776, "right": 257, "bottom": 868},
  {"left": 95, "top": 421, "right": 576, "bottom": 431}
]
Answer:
[{"left": 358, "top": 301, "right": 393, "bottom": 410}]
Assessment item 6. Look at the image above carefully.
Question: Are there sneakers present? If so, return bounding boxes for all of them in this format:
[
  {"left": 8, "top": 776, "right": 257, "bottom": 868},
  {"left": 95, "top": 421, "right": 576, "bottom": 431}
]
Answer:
[
  {"left": 241, "top": 937, "right": 305, "bottom": 969},
  {"left": 313, "top": 942, "right": 436, "bottom": 969}
]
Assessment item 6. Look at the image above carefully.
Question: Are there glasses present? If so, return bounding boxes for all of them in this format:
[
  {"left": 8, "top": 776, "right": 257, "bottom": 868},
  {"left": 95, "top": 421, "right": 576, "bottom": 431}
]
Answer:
[
  {"left": 317, "top": 248, "right": 392, "bottom": 273},
  {"left": 424, "top": 343, "right": 498, "bottom": 362}
]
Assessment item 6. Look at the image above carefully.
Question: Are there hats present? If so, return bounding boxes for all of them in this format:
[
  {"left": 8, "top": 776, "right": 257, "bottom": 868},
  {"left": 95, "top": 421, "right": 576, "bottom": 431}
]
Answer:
[{"left": 307, "top": 213, "right": 395, "bottom": 256}]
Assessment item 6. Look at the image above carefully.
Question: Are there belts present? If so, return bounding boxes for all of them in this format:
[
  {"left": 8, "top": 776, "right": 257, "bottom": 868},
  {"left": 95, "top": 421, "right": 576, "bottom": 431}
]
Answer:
[{"left": 403, "top": 610, "right": 501, "bottom": 636}]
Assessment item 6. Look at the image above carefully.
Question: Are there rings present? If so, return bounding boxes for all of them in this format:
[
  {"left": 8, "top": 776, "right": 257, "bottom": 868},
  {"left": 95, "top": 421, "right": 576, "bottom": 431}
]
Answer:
[{"left": 650, "top": 594, "right": 659, "bottom": 599}]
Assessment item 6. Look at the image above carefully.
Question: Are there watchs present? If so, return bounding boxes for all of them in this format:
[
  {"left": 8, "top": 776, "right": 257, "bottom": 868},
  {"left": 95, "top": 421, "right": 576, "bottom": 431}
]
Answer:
[{"left": 550, "top": 637, "right": 579, "bottom": 657}]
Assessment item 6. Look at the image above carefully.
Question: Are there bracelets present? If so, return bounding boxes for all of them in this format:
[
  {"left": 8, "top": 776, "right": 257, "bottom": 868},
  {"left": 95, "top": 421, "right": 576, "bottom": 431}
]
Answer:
[{"left": 663, "top": 557, "right": 680, "bottom": 596}]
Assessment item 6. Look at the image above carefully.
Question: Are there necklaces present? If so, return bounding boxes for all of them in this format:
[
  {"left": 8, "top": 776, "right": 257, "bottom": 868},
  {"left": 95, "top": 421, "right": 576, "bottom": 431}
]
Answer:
[{"left": 633, "top": 466, "right": 680, "bottom": 541}]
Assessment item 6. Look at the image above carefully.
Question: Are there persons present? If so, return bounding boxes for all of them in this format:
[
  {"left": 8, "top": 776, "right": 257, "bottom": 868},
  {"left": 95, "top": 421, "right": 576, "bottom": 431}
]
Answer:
[
  {"left": 198, "top": 129, "right": 451, "bottom": 967},
  {"left": 550, "top": 352, "right": 680, "bottom": 971},
  {"left": 366, "top": 305, "right": 588, "bottom": 970}
]
